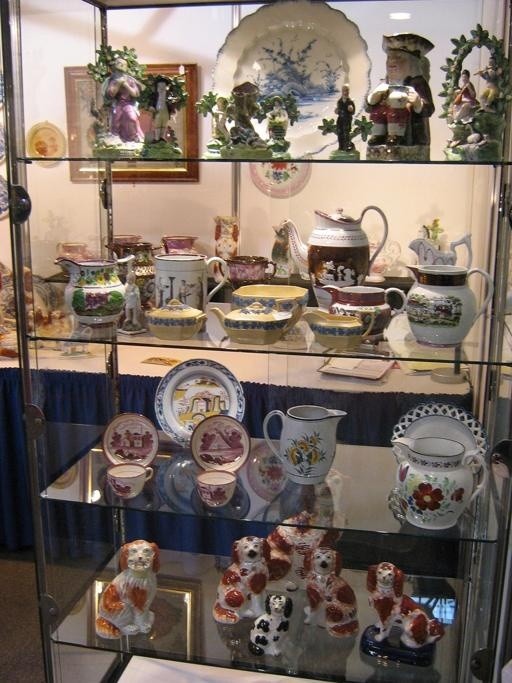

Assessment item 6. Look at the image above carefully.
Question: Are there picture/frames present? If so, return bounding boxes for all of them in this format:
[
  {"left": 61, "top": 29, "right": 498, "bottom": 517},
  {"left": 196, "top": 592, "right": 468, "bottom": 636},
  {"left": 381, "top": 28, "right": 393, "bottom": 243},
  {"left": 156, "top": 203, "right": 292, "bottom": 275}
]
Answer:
[{"left": 60, "top": 61, "right": 202, "bottom": 188}]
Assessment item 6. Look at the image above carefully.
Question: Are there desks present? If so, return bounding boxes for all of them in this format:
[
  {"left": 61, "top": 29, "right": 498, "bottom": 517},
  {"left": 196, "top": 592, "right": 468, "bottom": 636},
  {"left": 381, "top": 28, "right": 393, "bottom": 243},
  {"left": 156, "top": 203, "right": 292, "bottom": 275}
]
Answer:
[{"left": 0, "top": 302, "right": 474, "bottom": 578}]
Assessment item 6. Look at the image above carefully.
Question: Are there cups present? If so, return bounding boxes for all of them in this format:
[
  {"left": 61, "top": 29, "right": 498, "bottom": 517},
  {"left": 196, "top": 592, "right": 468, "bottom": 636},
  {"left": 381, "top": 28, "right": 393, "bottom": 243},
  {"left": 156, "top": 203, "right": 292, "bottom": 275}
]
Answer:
[
  {"left": 314, "top": 468, "right": 353, "bottom": 506},
  {"left": 272, "top": 226, "right": 294, "bottom": 280},
  {"left": 221, "top": 257, "right": 279, "bottom": 291},
  {"left": 124, "top": 243, "right": 163, "bottom": 268},
  {"left": 367, "top": 236, "right": 404, "bottom": 283},
  {"left": 57, "top": 242, "right": 90, "bottom": 263},
  {"left": 213, "top": 215, "right": 241, "bottom": 286}
]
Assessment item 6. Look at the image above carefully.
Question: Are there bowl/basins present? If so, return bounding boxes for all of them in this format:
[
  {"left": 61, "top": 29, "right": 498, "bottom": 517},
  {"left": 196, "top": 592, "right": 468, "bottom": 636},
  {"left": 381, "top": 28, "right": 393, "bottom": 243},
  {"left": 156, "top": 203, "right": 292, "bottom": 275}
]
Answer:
[
  {"left": 230, "top": 284, "right": 309, "bottom": 333},
  {"left": 195, "top": 470, "right": 237, "bottom": 509},
  {"left": 108, "top": 464, "right": 154, "bottom": 499}
]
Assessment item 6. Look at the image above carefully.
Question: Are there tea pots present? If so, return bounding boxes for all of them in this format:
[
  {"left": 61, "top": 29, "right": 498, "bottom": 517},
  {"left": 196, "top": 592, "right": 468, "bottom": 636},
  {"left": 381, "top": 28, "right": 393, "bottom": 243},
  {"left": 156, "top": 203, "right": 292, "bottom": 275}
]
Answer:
[
  {"left": 104, "top": 239, "right": 163, "bottom": 310},
  {"left": 112, "top": 234, "right": 143, "bottom": 243},
  {"left": 143, "top": 295, "right": 209, "bottom": 340},
  {"left": 262, "top": 405, "right": 346, "bottom": 488},
  {"left": 409, "top": 234, "right": 474, "bottom": 272},
  {"left": 390, "top": 432, "right": 484, "bottom": 528},
  {"left": 159, "top": 230, "right": 198, "bottom": 255},
  {"left": 280, "top": 205, "right": 388, "bottom": 314},
  {"left": 210, "top": 301, "right": 299, "bottom": 347},
  {"left": 53, "top": 257, "right": 133, "bottom": 342},
  {"left": 301, "top": 307, "right": 377, "bottom": 360},
  {"left": 152, "top": 252, "right": 230, "bottom": 331},
  {"left": 319, "top": 282, "right": 407, "bottom": 344},
  {"left": 400, "top": 260, "right": 491, "bottom": 347}
]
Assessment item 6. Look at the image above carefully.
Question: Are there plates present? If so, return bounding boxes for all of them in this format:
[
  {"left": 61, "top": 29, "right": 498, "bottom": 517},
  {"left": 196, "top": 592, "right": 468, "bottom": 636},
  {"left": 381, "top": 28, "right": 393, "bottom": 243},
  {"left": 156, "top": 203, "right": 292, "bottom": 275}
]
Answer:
[
  {"left": 316, "top": 351, "right": 399, "bottom": 382},
  {"left": 1, "top": 275, "right": 58, "bottom": 335},
  {"left": 25, "top": 122, "right": 67, "bottom": 167},
  {"left": 154, "top": 358, "right": 247, "bottom": 451},
  {"left": 103, "top": 413, "right": 160, "bottom": 467},
  {"left": 156, "top": 453, "right": 205, "bottom": 515},
  {"left": 52, "top": 462, "right": 80, "bottom": 490},
  {"left": 190, "top": 415, "right": 250, "bottom": 472},
  {"left": 246, "top": 441, "right": 290, "bottom": 503},
  {"left": 189, "top": 483, "right": 252, "bottom": 520},
  {"left": 390, "top": 401, "right": 488, "bottom": 475},
  {"left": 212, "top": 0, "right": 371, "bottom": 164},
  {"left": 246, "top": 161, "right": 311, "bottom": 198},
  {"left": 0, "top": 123, "right": 7, "bottom": 164},
  {"left": 1, "top": 175, "right": 11, "bottom": 222}
]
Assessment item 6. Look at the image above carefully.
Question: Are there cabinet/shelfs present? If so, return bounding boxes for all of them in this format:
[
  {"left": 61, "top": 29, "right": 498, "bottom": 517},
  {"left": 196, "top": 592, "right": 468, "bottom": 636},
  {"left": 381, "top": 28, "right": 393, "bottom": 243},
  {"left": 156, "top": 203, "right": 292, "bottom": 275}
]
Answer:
[{"left": 0, "top": 0, "right": 511, "bottom": 683}]
